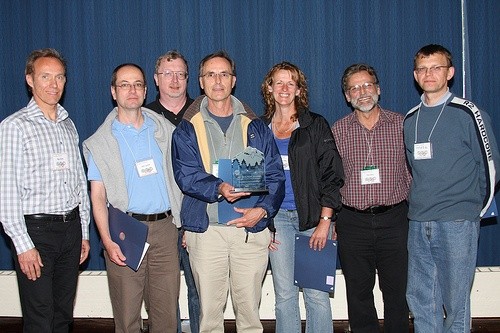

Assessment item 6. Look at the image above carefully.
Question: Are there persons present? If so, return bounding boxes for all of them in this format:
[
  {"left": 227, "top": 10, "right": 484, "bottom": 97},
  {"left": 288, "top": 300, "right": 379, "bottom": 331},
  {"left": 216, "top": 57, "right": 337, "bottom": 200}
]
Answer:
[
  {"left": 403, "top": 44, "right": 500, "bottom": 333},
  {"left": 0, "top": 48, "right": 91, "bottom": 333},
  {"left": 171, "top": 52, "right": 286, "bottom": 333},
  {"left": 256, "top": 62, "right": 346, "bottom": 333},
  {"left": 146, "top": 49, "right": 200, "bottom": 333},
  {"left": 330, "top": 64, "right": 412, "bottom": 333},
  {"left": 83, "top": 63, "right": 183, "bottom": 333}
]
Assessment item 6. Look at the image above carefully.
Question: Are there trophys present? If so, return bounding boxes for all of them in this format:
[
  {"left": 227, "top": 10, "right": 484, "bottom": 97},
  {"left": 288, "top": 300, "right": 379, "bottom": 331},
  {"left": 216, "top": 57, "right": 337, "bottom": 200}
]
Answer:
[{"left": 229, "top": 147, "right": 268, "bottom": 193}]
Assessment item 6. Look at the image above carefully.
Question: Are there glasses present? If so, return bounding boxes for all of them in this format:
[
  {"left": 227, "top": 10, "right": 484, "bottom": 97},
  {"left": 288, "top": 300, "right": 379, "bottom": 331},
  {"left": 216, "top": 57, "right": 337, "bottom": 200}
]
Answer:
[
  {"left": 201, "top": 72, "right": 234, "bottom": 79},
  {"left": 346, "top": 82, "right": 378, "bottom": 93},
  {"left": 415, "top": 64, "right": 449, "bottom": 74},
  {"left": 113, "top": 83, "right": 144, "bottom": 90},
  {"left": 157, "top": 70, "right": 187, "bottom": 80}
]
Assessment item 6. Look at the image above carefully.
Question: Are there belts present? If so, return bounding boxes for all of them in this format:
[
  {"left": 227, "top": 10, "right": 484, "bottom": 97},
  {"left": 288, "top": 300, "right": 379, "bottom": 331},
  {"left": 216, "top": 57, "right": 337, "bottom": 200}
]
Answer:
[
  {"left": 129, "top": 210, "right": 171, "bottom": 221},
  {"left": 24, "top": 206, "right": 79, "bottom": 223},
  {"left": 341, "top": 202, "right": 403, "bottom": 215}
]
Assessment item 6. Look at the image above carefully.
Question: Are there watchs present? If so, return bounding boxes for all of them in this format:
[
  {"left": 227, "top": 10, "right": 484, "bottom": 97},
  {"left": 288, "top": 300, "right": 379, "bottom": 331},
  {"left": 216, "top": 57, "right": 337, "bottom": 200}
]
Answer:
[{"left": 320, "top": 216, "right": 332, "bottom": 221}]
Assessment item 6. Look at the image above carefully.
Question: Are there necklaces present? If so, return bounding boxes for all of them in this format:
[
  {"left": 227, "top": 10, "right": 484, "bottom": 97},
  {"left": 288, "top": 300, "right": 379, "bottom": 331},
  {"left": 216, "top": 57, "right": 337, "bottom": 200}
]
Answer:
[{"left": 274, "top": 116, "right": 296, "bottom": 134}]
